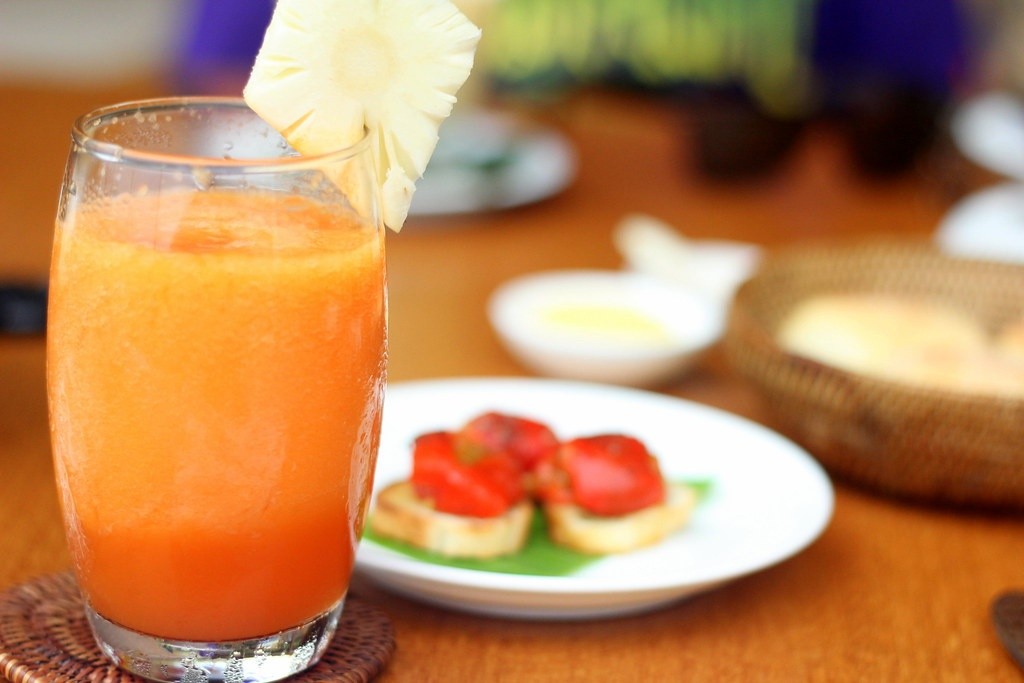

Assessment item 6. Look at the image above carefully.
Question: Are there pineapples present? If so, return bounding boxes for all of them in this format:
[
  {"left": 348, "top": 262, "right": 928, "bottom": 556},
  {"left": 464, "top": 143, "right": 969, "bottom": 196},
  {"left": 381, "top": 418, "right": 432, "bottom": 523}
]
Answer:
[{"left": 242, "top": 0, "right": 483, "bottom": 241}]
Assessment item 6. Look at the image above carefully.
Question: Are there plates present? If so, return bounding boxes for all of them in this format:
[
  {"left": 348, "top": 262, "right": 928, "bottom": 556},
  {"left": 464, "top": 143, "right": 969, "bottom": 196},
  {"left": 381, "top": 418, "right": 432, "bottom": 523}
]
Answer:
[
  {"left": 352, "top": 372, "right": 841, "bottom": 626},
  {"left": 207, "top": 103, "right": 578, "bottom": 232},
  {"left": 485, "top": 261, "right": 726, "bottom": 390}
]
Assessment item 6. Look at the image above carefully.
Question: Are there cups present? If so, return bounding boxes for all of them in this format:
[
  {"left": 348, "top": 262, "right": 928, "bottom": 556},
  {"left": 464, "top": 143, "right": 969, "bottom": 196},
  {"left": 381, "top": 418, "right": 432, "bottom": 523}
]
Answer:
[{"left": 47, "top": 94, "right": 390, "bottom": 681}]
